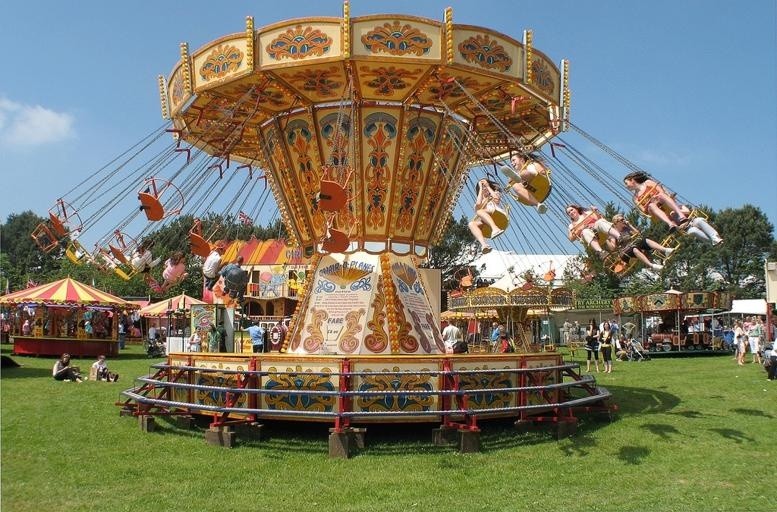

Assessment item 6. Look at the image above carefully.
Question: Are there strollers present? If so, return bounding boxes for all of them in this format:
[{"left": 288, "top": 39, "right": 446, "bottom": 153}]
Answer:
[
  {"left": 628, "top": 338, "right": 650, "bottom": 363},
  {"left": 144, "top": 338, "right": 167, "bottom": 358}
]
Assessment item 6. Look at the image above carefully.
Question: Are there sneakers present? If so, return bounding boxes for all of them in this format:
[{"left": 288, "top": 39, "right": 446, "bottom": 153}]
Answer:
[
  {"left": 594, "top": 216, "right": 724, "bottom": 272},
  {"left": 538, "top": 205, "right": 547, "bottom": 214},
  {"left": 502, "top": 166, "right": 522, "bottom": 183}
]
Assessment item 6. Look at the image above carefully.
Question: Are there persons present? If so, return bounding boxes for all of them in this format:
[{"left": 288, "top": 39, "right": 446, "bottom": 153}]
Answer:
[
  {"left": 562, "top": 317, "right": 636, "bottom": 373},
  {"left": 203, "top": 246, "right": 251, "bottom": 304},
  {"left": 500, "top": 151, "right": 548, "bottom": 215},
  {"left": 670, "top": 204, "right": 724, "bottom": 249},
  {"left": 469, "top": 179, "right": 504, "bottom": 253},
  {"left": 687, "top": 313, "right": 777, "bottom": 382},
  {"left": 442, "top": 318, "right": 461, "bottom": 353},
  {"left": 623, "top": 172, "right": 688, "bottom": 234},
  {"left": 607, "top": 213, "right": 675, "bottom": 272},
  {"left": 488, "top": 319, "right": 513, "bottom": 354},
  {"left": 566, "top": 205, "right": 623, "bottom": 261},
  {"left": 0, "top": 302, "right": 228, "bottom": 384},
  {"left": 160, "top": 251, "right": 190, "bottom": 290},
  {"left": 132, "top": 237, "right": 161, "bottom": 273},
  {"left": 240, "top": 320, "right": 266, "bottom": 352}
]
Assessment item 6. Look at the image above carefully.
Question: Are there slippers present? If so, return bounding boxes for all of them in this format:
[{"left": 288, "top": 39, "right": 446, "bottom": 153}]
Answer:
[
  {"left": 482, "top": 246, "right": 493, "bottom": 254},
  {"left": 491, "top": 230, "right": 505, "bottom": 240}
]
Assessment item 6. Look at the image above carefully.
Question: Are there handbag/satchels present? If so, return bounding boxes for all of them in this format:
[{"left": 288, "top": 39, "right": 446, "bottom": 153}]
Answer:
[{"left": 88, "top": 367, "right": 99, "bottom": 381}]
